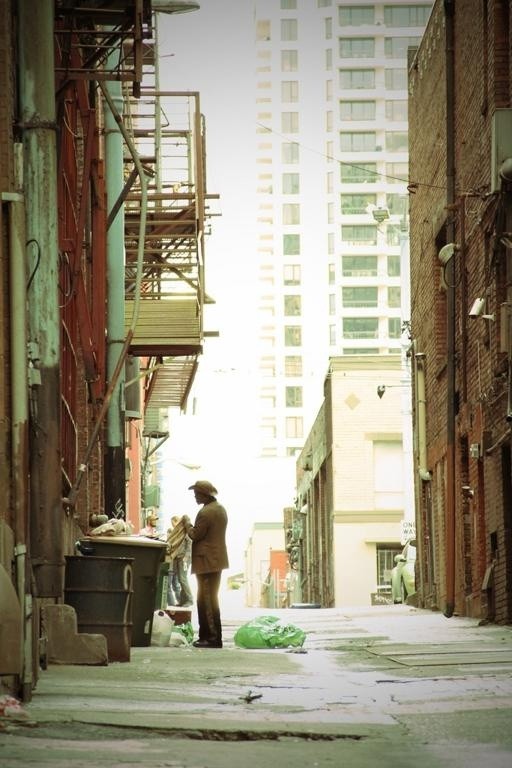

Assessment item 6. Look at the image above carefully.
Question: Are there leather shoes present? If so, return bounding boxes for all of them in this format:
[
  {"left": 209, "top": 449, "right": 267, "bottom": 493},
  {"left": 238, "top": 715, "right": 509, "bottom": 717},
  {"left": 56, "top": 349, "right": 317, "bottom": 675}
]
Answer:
[{"left": 192, "top": 638, "right": 222, "bottom": 648}]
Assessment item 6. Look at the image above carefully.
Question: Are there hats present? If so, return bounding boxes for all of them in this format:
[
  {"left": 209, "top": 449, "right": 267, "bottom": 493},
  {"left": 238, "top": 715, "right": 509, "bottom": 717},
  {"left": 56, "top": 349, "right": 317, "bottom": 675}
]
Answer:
[
  {"left": 147, "top": 515, "right": 159, "bottom": 521},
  {"left": 187, "top": 480, "right": 219, "bottom": 496}
]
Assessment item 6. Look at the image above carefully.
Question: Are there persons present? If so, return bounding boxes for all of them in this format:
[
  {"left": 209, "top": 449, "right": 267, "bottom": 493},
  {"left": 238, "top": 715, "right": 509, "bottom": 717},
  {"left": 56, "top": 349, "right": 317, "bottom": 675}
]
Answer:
[
  {"left": 167, "top": 528, "right": 183, "bottom": 606},
  {"left": 137, "top": 515, "right": 160, "bottom": 540},
  {"left": 171, "top": 516, "right": 194, "bottom": 608},
  {"left": 181, "top": 479, "right": 230, "bottom": 648}
]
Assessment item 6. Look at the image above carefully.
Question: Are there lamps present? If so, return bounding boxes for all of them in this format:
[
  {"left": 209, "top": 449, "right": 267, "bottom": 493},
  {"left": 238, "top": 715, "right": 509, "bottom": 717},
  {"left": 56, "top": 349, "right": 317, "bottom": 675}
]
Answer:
[{"left": 378, "top": 382, "right": 415, "bottom": 398}]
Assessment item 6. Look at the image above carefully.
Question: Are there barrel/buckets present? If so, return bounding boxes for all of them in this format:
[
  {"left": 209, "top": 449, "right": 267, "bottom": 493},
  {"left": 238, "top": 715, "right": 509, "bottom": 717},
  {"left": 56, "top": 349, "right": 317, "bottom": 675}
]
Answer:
[{"left": 62, "top": 555, "right": 136, "bottom": 663}]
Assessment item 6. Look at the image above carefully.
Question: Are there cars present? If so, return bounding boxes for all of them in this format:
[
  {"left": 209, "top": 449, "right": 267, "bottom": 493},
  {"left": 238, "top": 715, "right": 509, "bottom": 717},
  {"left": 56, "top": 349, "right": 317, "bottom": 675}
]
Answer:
[{"left": 390, "top": 538, "right": 415, "bottom": 602}]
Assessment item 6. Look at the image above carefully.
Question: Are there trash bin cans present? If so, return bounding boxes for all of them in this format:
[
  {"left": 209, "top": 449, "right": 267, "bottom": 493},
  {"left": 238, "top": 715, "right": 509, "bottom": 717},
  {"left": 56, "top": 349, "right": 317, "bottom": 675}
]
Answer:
[{"left": 64, "top": 536, "right": 168, "bottom": 663}]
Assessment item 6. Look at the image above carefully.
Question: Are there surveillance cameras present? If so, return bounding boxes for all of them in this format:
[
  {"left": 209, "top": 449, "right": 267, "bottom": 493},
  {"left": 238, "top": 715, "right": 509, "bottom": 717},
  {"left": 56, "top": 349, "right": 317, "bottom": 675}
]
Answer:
[{"left": 467, "top": 297, "right": 486, "bottom": 319}]
